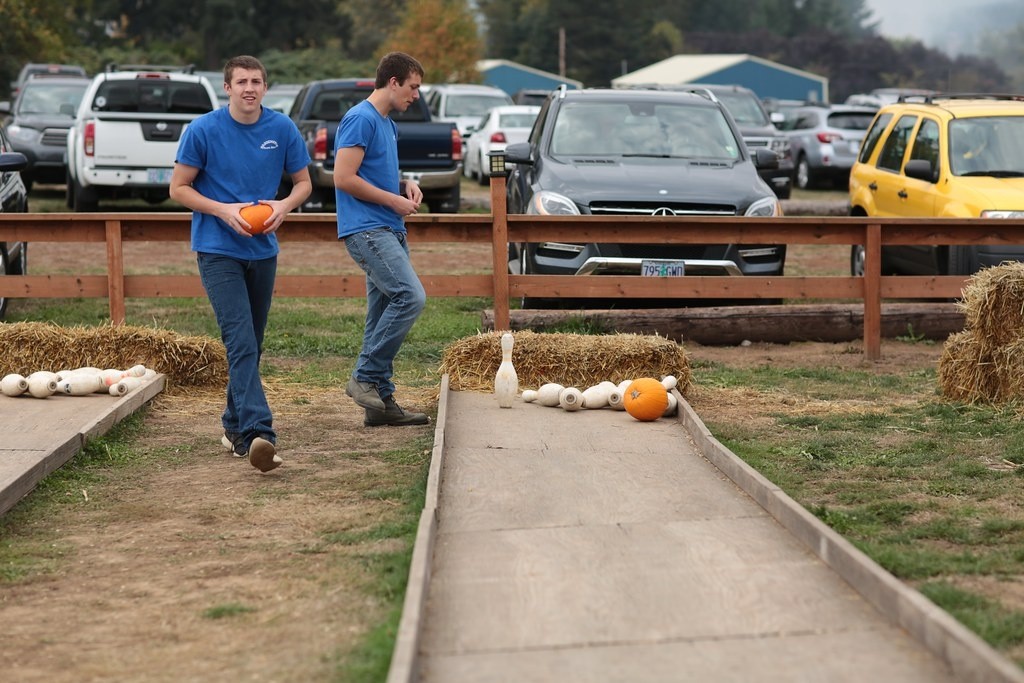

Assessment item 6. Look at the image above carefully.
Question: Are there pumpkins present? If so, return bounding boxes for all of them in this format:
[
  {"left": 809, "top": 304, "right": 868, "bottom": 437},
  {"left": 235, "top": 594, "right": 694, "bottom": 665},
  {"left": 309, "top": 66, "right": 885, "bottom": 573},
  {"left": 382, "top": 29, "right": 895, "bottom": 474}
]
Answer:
[
  {"left": 623, "top": 377, "right": 668, "bottom": 421},
  {"left": 238, "top": 204, "right": 276, "bottom": 234}
]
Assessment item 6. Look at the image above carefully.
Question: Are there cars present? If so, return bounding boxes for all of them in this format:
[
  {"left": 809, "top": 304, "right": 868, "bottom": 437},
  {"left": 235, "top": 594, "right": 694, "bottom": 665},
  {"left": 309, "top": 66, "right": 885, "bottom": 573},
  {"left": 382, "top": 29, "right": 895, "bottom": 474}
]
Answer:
[
  {"left": 0, "top": 78, "right": 93, "bottom": 191},
  {"left": 425, "top": 83, "right": 516, "bottom": 159},
  {"left": 9, "top": 63, "right": 88, "bottom": 111},
  {"left": 764, "top": 98, "right": 806, "bottom": 130},
  {"left": 512, "top": 89, "right": 552, "bottom": 106},
  {"left": 640, "top": 84, "right": 794, "bottom": 199},
  {"left": 463, "top": 105, "right": 542, "bottom": 186},
  {"left": 152, "top": 71, "right": 231, "bottom": 110},
  {"left": 0, "top": 126, "right": 29, "bottom": 317},
  {"left": 262, "top": 82, "right": 303, "bottom": 116}
]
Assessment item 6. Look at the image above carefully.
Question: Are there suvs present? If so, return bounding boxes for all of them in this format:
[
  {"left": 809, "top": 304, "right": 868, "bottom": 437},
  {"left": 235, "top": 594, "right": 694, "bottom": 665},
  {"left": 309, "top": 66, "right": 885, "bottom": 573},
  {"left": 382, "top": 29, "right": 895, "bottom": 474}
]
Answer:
[
  {"left": 504, "top": 84, "right": 787, "bottom": 310},
  {"left": 850, "top": 92, "right": 1024, "bottom": 276},
  {"left": 783, "top": 101, "right": 882, "bottom": 190}
]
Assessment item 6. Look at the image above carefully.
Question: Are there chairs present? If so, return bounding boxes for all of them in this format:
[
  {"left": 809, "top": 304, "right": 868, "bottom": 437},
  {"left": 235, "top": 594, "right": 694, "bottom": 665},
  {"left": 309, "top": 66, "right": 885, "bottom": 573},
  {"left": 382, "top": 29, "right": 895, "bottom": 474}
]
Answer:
[
  {"left": 318, "top": 97, "right": 341, "bottom": 119},
  {"left": 100, "top": 88, "right": 139, "bottom": 111},
  {"left": 168, "top": 89, "right": 199, "bottom": 112}
]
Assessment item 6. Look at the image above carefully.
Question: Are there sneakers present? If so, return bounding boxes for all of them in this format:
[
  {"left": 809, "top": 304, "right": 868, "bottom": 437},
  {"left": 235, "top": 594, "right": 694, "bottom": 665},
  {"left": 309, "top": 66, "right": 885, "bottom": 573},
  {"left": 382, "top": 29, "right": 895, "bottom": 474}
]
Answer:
[
  {"left": 247, "top": 426, "right": 283, "bottom": 472},
  {"left": 221, "top": 430, "right": 249, "bottom": 458},
  {"left": 346, "top": 374, "right": 386, "bottom": 414},
  {"left": 364, "top": 392, "right": 428, "bottom": 425}
]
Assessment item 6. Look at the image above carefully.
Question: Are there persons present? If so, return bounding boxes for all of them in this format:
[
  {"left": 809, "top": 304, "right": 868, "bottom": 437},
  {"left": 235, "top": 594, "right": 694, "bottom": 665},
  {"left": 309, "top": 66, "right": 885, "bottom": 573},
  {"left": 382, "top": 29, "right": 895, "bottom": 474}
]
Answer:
[
  {"left": 332, "top": 53, "right": 430, "bottom": 427},
  {"left": 169, "top": 56, "right": 314, "bottom": 473}
]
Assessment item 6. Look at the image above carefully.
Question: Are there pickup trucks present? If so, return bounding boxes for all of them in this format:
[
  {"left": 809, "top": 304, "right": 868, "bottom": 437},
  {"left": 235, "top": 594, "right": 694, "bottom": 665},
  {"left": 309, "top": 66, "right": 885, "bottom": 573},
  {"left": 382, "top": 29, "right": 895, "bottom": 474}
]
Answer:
[
  {"left": 271, "top": 78, "right": 468, "bottom": 214},
  {"left": 60, "top": 64, "right": 221, "bottom": 212}
]
